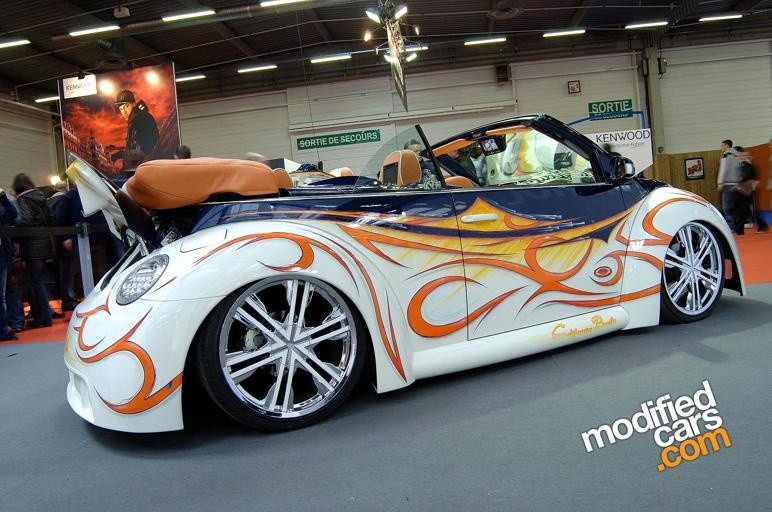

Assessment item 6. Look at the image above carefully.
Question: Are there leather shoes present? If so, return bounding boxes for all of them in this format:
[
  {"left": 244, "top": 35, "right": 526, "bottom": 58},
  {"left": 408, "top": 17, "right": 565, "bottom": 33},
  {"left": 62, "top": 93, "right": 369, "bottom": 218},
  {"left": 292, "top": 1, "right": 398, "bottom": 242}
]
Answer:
[{"left": 0, "top": 298, "right": 81, "bottom": 341}]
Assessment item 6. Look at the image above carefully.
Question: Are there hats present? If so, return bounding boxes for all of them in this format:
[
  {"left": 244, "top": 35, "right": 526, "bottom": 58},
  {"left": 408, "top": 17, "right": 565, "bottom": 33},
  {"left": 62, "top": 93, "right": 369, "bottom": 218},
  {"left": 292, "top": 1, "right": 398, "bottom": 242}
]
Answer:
[{"left": 114, "top": 90, "right": 135, "bottom": 105}]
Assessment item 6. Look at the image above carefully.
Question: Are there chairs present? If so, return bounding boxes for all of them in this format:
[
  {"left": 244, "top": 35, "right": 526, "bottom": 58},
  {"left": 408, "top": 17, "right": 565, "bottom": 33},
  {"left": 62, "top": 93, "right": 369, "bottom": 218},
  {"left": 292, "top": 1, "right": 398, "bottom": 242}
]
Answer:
[{"left": 376, "top": 150, "right": 477, "bottom": 189}]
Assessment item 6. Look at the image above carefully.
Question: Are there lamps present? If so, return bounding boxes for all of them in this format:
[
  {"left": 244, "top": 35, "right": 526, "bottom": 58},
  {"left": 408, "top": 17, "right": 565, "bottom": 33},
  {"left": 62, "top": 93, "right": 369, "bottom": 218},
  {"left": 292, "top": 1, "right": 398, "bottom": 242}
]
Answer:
[{"left": 362, "top": 0, "right": 423, "bottom": 66}]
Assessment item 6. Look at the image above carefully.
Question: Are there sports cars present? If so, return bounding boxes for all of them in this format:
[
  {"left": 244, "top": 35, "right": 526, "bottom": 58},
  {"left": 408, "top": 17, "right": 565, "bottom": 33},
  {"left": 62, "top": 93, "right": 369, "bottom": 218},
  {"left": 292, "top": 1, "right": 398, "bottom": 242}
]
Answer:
[{"left": 63, "top": 112, "right": 747, "bottom": 436}]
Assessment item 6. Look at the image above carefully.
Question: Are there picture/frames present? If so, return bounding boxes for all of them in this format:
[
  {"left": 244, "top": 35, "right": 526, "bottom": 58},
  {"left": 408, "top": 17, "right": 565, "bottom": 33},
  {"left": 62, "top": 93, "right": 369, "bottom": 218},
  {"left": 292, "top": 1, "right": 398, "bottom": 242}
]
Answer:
[{"left": 684, "top": 156, "right": 705, "bottom": 181}]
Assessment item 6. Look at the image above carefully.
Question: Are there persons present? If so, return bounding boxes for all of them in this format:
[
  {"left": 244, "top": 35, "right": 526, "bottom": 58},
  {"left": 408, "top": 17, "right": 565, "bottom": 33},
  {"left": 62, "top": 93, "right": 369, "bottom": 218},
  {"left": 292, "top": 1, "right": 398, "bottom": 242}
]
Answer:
[
  {"left": 448, "top": 147, "right": 479, "bottom": 182},
  {"left": 404, "top": 138, "right": 436, "bottom": 177},
  {"left": 0, "top": 172, "right": 128, "bottom": 344},
  {"left": 173, "top": 143, "right": 191, "bottom": 159},
  {"left": 715, "top": 139, "right": 770, "bottom": 235},
  {"left": 105, "top": 89, "right": 160, "bottom": 170}
]
